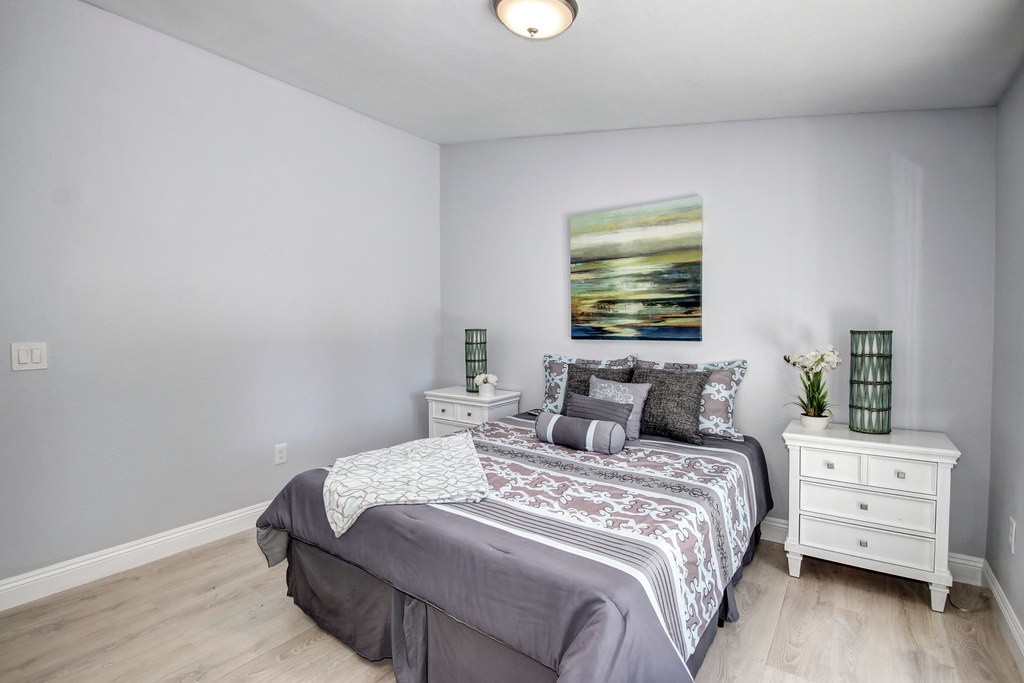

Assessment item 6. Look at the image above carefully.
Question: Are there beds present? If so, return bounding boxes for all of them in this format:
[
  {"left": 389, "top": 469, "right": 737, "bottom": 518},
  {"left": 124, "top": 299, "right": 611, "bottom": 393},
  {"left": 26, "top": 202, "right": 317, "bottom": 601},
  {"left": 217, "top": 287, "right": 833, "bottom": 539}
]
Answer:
[{"left": 255, "top": 408, "right": 774, "bottom": 683}]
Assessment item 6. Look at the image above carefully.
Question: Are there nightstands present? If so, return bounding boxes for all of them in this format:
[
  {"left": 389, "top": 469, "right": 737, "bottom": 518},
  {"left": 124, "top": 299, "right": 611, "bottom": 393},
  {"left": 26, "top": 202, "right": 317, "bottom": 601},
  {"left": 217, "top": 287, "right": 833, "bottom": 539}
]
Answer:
[{"left": 782, "top": 419, "right": 962, "bottom": 611}]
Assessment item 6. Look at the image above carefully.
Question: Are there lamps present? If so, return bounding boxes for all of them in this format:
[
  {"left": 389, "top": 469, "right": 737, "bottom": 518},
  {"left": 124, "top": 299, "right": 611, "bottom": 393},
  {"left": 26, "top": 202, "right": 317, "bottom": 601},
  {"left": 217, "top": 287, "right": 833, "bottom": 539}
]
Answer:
[
  {"left": 464, "top": 328, "right": 488, "bottom": 392},
  {"left": 849, "top": 329, "right": 894, "bottom": 434},
  {"left": 494, "top": 0, "right": 579, "bottom": 39}
]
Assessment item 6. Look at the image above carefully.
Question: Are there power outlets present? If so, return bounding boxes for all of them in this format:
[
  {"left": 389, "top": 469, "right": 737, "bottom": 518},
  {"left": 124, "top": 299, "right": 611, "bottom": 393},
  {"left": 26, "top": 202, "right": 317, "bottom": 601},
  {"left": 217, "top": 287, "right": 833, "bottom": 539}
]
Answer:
[
  {"left": 275, "top": 444, "right": 289, "bottom": 464},
  {"left": 1007, "top": 517, "right": 1015, "bottom": 553}
]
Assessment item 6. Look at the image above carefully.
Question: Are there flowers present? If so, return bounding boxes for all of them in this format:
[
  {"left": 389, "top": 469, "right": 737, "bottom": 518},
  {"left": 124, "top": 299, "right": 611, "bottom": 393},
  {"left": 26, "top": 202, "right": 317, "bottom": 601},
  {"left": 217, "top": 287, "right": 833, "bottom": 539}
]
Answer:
[
  {"left": 475, "top": 373, "right": 498, "bottom": 386},
  {"left": 781, "top": 345, "right": 842, "bottom": 421}
]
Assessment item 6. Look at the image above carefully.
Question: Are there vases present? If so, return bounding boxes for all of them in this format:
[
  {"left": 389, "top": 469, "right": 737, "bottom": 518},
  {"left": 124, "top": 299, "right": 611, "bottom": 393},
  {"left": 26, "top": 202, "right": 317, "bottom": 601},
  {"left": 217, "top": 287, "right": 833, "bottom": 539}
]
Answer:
[
  {"left": 800, "top": 414, "right": 829, "bottom": 429},
  {"left": 479, "top": 384, "right": 494, "bottom": 396}
]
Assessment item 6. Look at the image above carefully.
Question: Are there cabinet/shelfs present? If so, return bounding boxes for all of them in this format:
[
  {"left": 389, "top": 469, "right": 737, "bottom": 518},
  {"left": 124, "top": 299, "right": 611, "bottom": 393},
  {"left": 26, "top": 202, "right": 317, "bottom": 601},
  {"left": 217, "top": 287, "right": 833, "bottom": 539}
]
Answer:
[{"left": 424, "top": 385, "right": 521, "bottom": 438}]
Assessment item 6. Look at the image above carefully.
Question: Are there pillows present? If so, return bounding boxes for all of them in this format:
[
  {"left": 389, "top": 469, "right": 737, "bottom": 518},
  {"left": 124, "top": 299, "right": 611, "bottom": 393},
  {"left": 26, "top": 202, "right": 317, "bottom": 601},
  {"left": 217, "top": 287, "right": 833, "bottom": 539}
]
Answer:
[{"left": 534, "top": 354, "right": 747, "bottom": 455}]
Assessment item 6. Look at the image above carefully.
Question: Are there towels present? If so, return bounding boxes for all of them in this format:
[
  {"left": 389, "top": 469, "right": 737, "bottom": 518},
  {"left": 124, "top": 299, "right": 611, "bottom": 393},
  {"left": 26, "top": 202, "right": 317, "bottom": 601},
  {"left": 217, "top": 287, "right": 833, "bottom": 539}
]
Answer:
[{"left": 323, "top": 432, "right": 489, "bottom": 538}]
